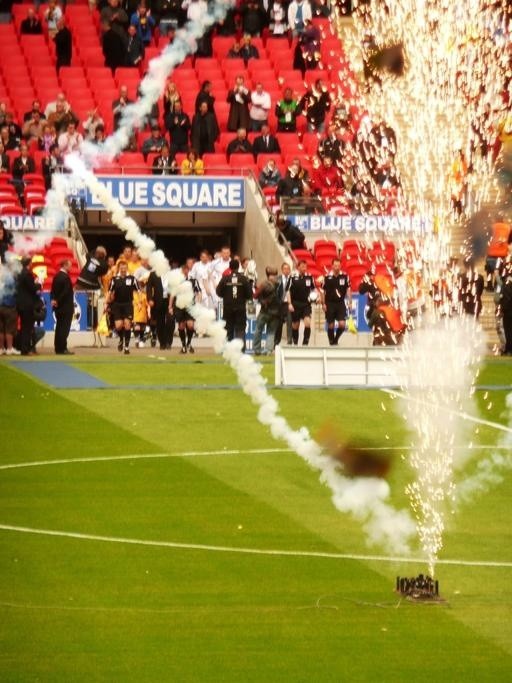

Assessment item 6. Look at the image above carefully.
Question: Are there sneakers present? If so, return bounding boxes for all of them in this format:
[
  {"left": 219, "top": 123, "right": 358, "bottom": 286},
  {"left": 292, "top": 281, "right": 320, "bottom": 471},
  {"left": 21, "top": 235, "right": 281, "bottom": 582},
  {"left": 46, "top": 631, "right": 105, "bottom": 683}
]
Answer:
[
  {"left": 118, "top": 339, "right": 194, "bottom": 355},
  {"left": 0, "top": 348, "right": 21, "bottom": 356}
]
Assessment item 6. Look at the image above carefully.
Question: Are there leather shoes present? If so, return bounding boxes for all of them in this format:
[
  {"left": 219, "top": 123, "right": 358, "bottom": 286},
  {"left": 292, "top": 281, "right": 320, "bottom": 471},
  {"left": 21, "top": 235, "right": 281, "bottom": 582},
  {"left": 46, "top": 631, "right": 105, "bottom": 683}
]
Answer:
[
  {"left": 56, "top": 349, "right": 75, "bottom": 355},
  {"left": 26, "top": 351, "right": 40, "bottom": 355}
]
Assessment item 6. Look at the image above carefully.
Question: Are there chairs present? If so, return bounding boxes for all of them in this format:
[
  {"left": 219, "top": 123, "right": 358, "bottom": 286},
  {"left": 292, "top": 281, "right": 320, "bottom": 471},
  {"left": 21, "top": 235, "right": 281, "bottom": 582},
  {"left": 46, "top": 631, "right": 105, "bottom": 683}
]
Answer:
[{"left": 0, "top": 0, "right": 415, "bottom": 295}]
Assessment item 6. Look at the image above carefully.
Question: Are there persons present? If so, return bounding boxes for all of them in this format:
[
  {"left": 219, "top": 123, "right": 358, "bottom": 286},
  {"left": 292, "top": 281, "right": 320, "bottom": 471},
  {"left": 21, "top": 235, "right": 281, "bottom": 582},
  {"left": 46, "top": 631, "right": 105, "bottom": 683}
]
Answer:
[{"left": 0, "top": 2, "right": 511, "bottom": 358}]
